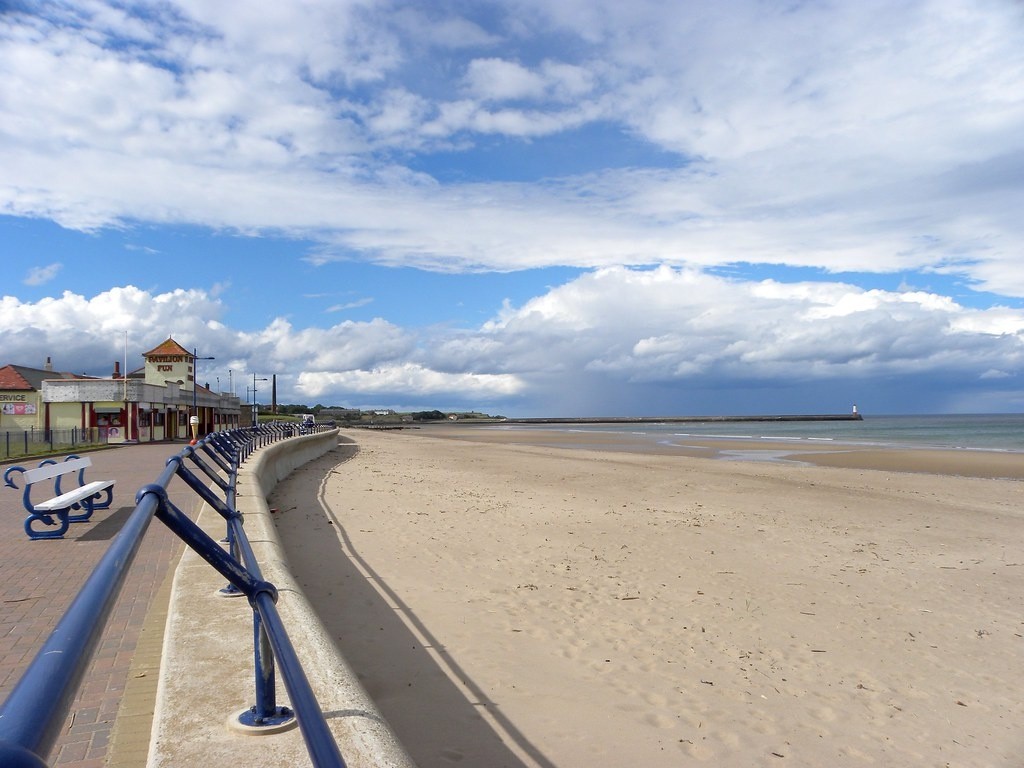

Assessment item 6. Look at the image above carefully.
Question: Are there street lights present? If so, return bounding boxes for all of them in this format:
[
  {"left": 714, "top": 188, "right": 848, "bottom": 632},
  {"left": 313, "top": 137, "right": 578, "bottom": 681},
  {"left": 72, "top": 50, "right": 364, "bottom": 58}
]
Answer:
[
  {"left": 247, "top": 386, "right": 258, "bottom": 403},
  {"left": 254, "top": 372, "right": 268, "bottom": 426},
  {"left": 193, "top": 348, "right": 215, "bottom": 416}
]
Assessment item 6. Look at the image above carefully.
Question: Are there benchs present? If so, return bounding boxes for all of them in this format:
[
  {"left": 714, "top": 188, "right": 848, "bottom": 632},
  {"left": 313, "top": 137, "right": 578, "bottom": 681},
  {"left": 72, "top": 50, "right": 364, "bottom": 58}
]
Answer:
[
  {"left": 4, "top": 455, "right": 116, "bottom": 541},
  {"left": 213, "top": 433, "right": 237, "bottom": 453},
  {"left": 299, "top": 426, "right": 307, "bottom": 435}
]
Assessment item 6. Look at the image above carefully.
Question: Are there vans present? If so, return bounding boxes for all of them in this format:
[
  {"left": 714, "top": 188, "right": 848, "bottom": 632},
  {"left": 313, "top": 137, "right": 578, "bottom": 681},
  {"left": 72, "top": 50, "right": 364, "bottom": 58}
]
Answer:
[{"left": 302, "top": 414, "right": 315, "bottom": 425}]
